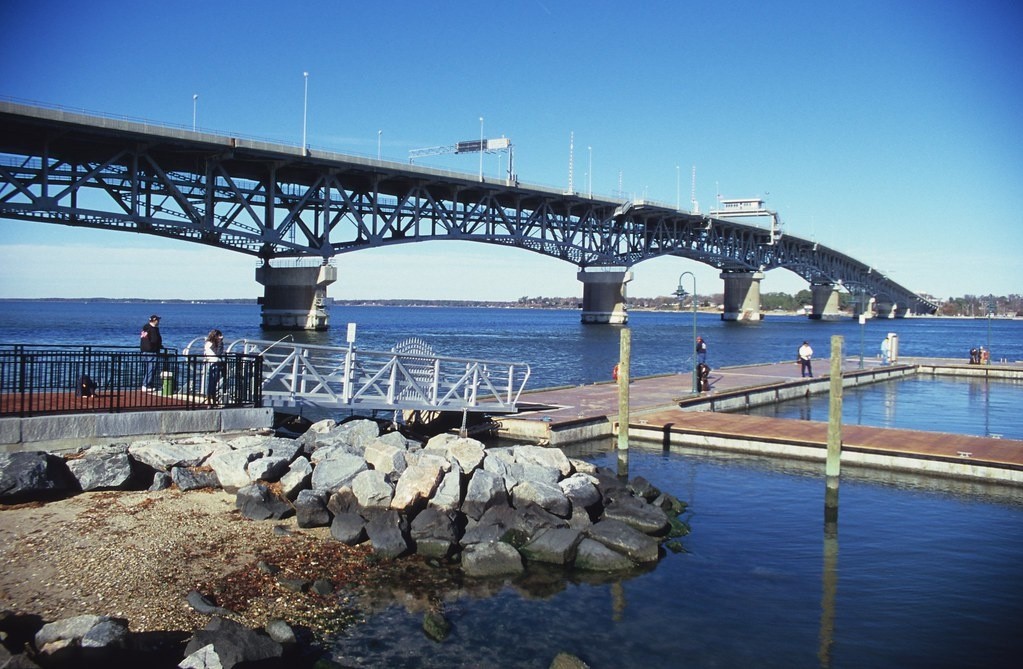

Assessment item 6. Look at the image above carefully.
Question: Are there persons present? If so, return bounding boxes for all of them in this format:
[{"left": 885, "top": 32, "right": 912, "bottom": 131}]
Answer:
[
  {"left": 881, "top": 338, "right": 890, "bottom": 365},
  {"left": 970, "top": 348, "right": 978, "bottom": 364},
  {"left": 696, "top": 337, "right": 707, "bottom": 365},
  {"left": 799, "top": 341, "right": 813, "bottom": 378},
  {"left": 205, "top": 329, "right": 225, "bottom": 408},
  {"left": 76, "top": 375, "right": 98, "bottom": 399},
  {"left": 979, "top": 346, "right": 987, "bottom": 365},
  {"left": 141, "top": 315, "right": 162, "bottom": 391}
]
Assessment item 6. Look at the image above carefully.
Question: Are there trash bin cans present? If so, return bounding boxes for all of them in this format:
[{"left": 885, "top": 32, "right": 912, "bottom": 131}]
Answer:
[
  {"left": 161, "top": 377, "right": 173, "bottom": 397},
  {"left": 887, "top": 332, "right": 898, "bottom": 366}
]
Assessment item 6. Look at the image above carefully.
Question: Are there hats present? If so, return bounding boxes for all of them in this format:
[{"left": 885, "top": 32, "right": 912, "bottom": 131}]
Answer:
[
  {"left": 804, "top": 342, "right": 809, "bottom": 344},
  {"left": 149, "top": 315, "right": 161, "bottom": 321}
]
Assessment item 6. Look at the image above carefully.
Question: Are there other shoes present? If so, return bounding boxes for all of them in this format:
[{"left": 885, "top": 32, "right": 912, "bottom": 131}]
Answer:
[
  {"left": 204, "top": 397, "right": 218, "bottom": 404},
  {"left": 809, "top": 373, "right": 812, "bottom": 377},
  {"left": 142, "top": 385, "right": 156, "bottom": 392},
  {"left": 803, "top": 374, "right": 805, "bottom": 377}
]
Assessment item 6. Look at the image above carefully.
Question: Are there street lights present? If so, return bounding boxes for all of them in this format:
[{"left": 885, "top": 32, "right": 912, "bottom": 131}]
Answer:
[
  {"left": 302, "top": 72, "right": 309, "bottom": 157},
  {"left": 676, "top": 166, "right": 680, "bottom": 209},
  {"left": 671, "top": 272, "right": 701, "bottom": 395},
  {"left": 844, "top": 285, "right": 865, "bottom": 370},
  {"left": 193, "top": 95, "right": 197, "bottom": 131},
  {"left": 588, "top": 146, "right": 593, "bottom": 198},
  {"left": 378, "top": 130, "right": 382, "bottom": 159},
  {"left": 498, "top": 155, "right": 502, "bottom": 180},
  {"left": 479, "top": 118, "right": 485, "bottom": 182}
]
